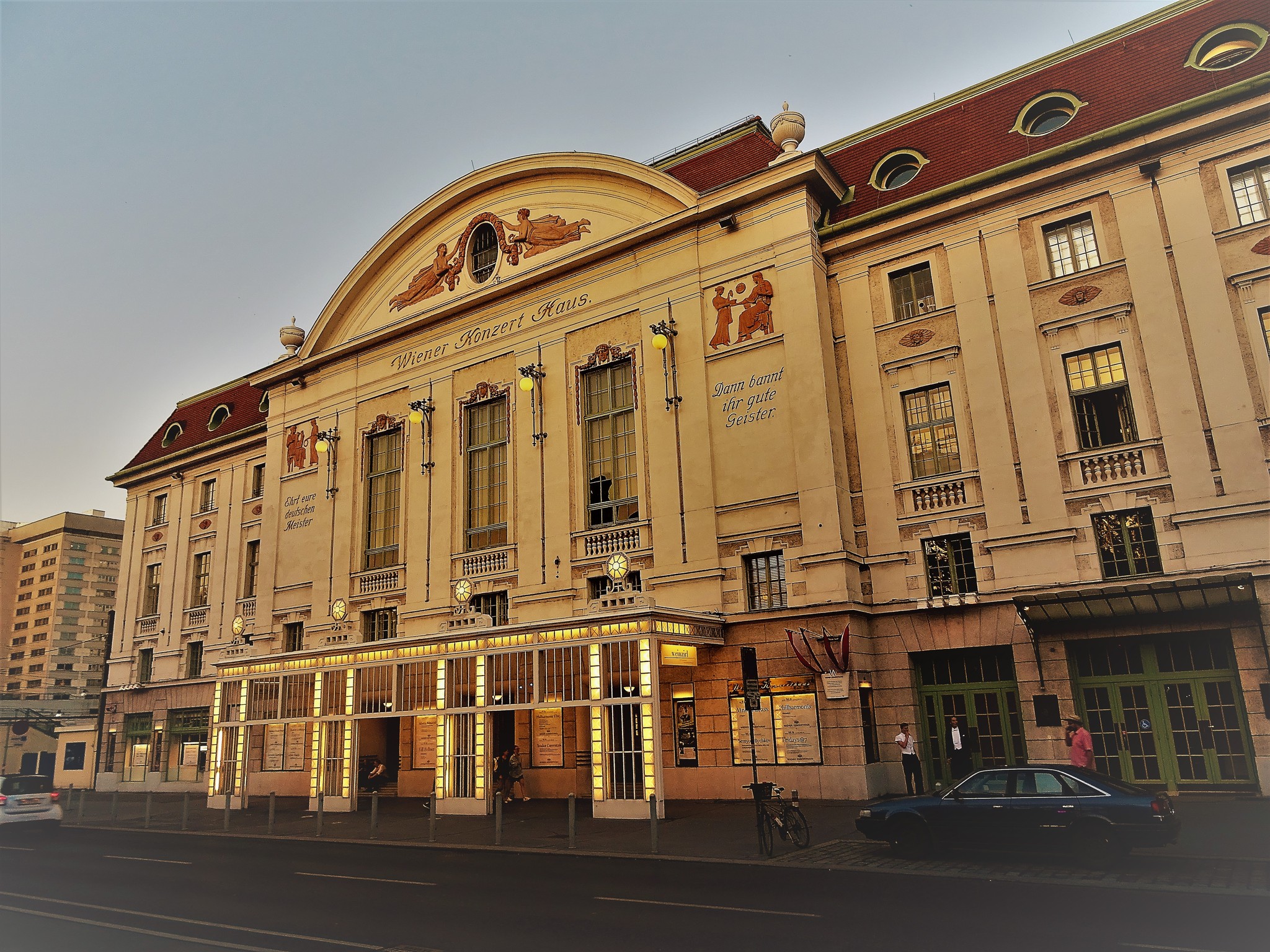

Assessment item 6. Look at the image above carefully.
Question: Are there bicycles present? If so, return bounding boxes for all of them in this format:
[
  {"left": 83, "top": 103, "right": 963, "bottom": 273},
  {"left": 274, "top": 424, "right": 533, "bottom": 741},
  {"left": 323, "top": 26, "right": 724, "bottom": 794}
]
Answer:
[{"left": 741, "top": 783, "right": 809, "bottom": 857}]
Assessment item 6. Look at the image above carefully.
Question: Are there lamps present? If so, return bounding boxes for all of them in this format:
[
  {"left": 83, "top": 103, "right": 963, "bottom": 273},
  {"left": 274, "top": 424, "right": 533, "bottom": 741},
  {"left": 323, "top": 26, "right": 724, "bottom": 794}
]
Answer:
[
  {"left": 383, "top": 702, "right": 393, "bottom": 708},
  {"left": 170, "top": 471, "right": 185, "bottom": 481},
  {"left": 623, "top": 686, "right": 635, "bottom": 693},
  {"left": 492, "top": 695, "right": 503, "bottom": 701},
  {"left": 291, "top": 377, "right": 306, "bottom": 387},
  {"left": 408, "top": 376, "right": 436, "bottom": 602},
  {"left": 1137, "top": 158, "right": 1163, "bottom": 175},
  {"left": 647, "top": 297, "right": 688, "bottom": 564},
  {"left": 314, "top": 407, "right": 342, "bottom": 616},
  {"left": 517, "top": 340, "right": 546, "bottom": 584},
  {"left": 718, "top": 213, "right": 737, "bottom": 230}
]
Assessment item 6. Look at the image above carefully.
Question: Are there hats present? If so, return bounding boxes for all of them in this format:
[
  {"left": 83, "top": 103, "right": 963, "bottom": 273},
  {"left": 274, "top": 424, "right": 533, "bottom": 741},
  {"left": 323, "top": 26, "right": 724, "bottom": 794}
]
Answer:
[{"left": 1061, "top": 714, "right": 1083, "bottom": 725}]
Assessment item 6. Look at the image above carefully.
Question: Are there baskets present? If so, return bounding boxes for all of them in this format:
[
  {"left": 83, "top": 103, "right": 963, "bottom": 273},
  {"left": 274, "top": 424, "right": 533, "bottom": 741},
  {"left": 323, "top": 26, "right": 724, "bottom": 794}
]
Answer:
[{"left": 750, "top": 783, "right": 773, "bottom": 799}]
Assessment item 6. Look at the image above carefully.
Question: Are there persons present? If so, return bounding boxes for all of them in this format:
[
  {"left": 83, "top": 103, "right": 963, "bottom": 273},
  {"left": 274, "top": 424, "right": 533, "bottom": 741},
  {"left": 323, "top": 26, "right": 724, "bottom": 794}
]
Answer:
[
  {"left": 361, "top": 759, "right": 387, "bottom": 793},
  {"left": 495, "top": 208, "right": 592, "bottom": 244},
  {"left": 596, "top": 344, "right": 610, "bottom": 364},
  {"left": 709, "top": 272, "right": 775, "bottom": 350},
  {"left": 389, "top": 233, "right": 464, "bottom": 313},
  {"left": 477, "top": 382, "right": 488, "bottom": 401},
  {"left": 286, "top": 419, "right": 319, "bottom": 474},
  {"left": 376, "top": 413, "right": 387, "bottom": 430},
  {"left": 945, "top": 716, "right": 972, "bottom": 780},
  {"left": 423, "top": 774, "right": 436, "bottom": 810},
  {"left": 895, "top": 723, "right": 925, "bottom": 796},
  {"left": 1061, "top": 714, "right": 1098, "bottom": 792},
  {"left": 492, "top": 745, "right": 530, "bottom": 803}
]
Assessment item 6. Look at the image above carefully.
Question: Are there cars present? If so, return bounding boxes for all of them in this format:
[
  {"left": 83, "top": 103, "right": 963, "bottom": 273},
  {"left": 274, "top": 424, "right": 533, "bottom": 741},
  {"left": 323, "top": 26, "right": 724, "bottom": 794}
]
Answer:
[
  {"left": 0, "top": 773, "right": 64, "bottom": 835},
  {"left": 856, "top": 763, "right": 1182, "bottom": 870}
]
